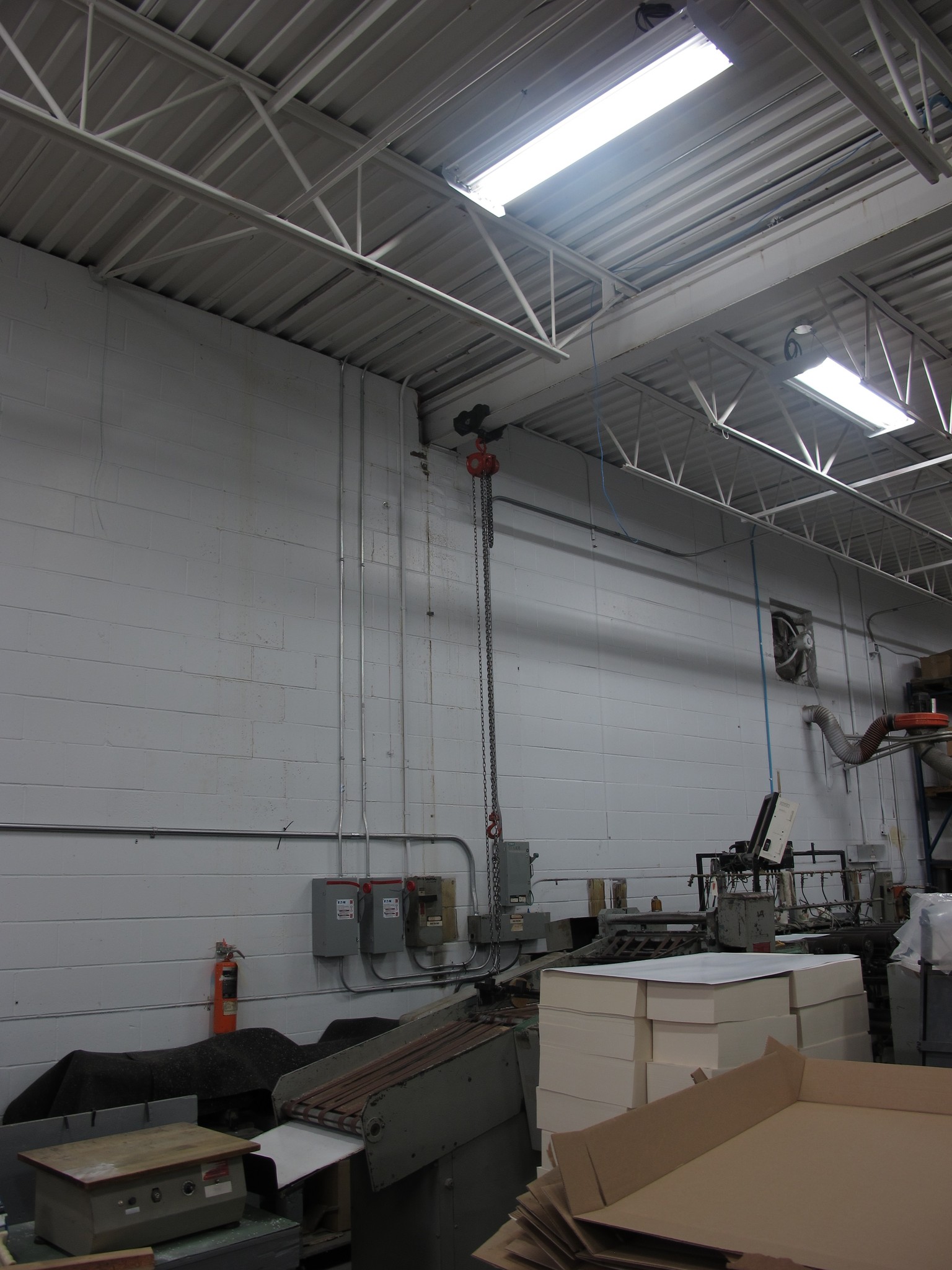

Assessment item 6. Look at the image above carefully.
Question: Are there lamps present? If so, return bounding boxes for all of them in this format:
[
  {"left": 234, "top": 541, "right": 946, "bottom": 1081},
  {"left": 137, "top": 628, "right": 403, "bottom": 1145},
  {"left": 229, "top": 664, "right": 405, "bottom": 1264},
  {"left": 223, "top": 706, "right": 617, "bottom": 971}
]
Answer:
[
  {"left": 774, "top": 317, "right": 915, "bottom": 441},
  {"left": 439, "top": 7, "right": 743, "bottom": 218}
]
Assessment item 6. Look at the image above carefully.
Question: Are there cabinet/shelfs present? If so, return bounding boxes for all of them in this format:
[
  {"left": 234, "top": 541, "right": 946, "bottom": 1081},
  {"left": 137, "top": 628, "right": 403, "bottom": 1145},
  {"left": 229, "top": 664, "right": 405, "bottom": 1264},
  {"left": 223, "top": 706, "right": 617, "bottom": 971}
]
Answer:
[{"left": 905, "top": 676, "right": 952, "bottom": 893}]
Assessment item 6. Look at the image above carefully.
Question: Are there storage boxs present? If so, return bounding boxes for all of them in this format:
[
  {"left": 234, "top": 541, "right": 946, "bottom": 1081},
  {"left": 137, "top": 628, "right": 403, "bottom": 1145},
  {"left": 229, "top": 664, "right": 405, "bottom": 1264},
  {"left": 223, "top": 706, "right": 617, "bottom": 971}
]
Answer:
[
  {"left": 919, "top": 649, "right": 952, "bottom": 796},
  {"left": 536, "top": 955, "right": 873, "bottom": 1182}
]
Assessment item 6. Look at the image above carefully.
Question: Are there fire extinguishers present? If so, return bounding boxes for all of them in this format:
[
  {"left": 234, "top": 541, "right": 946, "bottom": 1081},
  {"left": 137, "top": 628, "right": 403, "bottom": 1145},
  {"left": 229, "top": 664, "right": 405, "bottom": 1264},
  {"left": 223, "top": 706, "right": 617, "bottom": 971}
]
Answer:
[{"left": 213, "top": 938, "right": 245, "bottom": 1033}]
